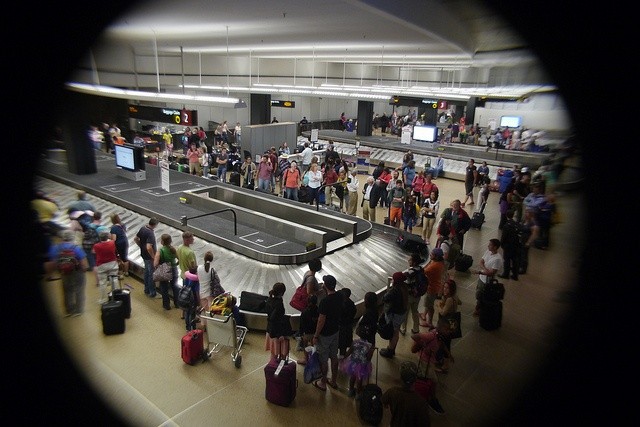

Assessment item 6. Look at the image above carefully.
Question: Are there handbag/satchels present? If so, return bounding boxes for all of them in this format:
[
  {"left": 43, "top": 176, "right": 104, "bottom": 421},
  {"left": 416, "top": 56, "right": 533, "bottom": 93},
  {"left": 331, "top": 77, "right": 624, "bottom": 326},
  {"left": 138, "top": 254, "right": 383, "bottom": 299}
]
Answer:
[
  {"left": 439, "top": 296, "right": 463, "bottom": 338},
  {"left": 377, "top": 287, "right": 394, "bottom": 340},
  {"left": 290, "top": 275, "right": 312, "bottom": 312},
  {"left": 483, "top": 280, "right": 504, "bottom": 298},
  {"left": 154, "top": 249, "right": 173, "bottom": 281},
  {"left": 210, "top": 267, "right": 224, "bottom": 298},
  {"left": 355, "top": 316, "right": 370, "bottom": 339},
  {"left": 304, "top": 337, "right": 323, "bottom": 383}
]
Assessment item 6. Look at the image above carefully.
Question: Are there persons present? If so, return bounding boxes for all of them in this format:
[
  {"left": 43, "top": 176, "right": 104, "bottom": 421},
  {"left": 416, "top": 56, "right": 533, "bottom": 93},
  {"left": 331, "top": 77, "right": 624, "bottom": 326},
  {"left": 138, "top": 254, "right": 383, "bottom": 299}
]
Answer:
[
  {"left": 372, "top": 161, "right": 385, "bottom": 181},
  {"left": 162, "top": 120, "right": 242, "bottom": 148},
  {"left": 436, "top": 199, "right": 472, "bottom": 271},
  {"left": 360, "top": 175, "right": 380, "bottom": 222},
  {"left": 257, "top": 155, "right": 274, "bottom": 192},
  {"left": 184, "top": 264, "right": 200, "bottom": 332},
  {"left": 199, "top": 147, "right": 211, "bottom": 178},
  {"left": 402, "top": 190, "right": 415, "bottom": 232},
  {"left": 266, "top": 149, "right": 278, "bottom": 193},
  {"left": 108, "top": 212, "right": 131, "bottom": 278},
  {"left": 269, "top": 146, "right": 277, "bottom": 156},
  {"left": 82, "top": 210, "right": 110, "bottom": 287},
  {"left": 477, "top": 183, "right": 491, "bottom": 224},
  {"left": 377, "top": 168, "right": 393, "bottom": 208},
  {"left": 186, "top": 143, "right": 201, "bottom": 176},
  {"left": 295, "top": 257, "right": 323, "bottom": 352},
  {"left": 134, "top": 216, "right": 160, "bottom": 298},
  {"left": 153, "top": 233, "right": 181, "bottom": 311},
  {"left": 346, "top": 325, "right": 373, "bottom": 401},
  {"left": 278, "top": 153, "right": 290, "bottom": 195},
  {"left": 279, "top": 141, "right": 290, "bottom": 156},
  {"left": 472, "top": 239, "right": 503, "bottom": 314},
  {"left": 216, "top": 148, "right": 228, "bottom": 183},
  {"left": 424, "top": 155, "right": 444, "bottom": 180},
  {"left": 321, "top": 159, "right": 347, "bottom": 214},
  {"left": 411, "top": 170, "right": 426, "bottom": 208},
  {"left": 66, "top": 207, "right": 96, "bottom": 241},
  {"left": 91, "top": 231, "right": 129, "bottom": 306},
  {"left": 439, "top": 229, "right": 452, "bottom": 264},
  {"left": 65, "top": 189, "right": 98, "bottom": 215},
  {"left": 388, "top": 180, "right": 405, "bottom": 228},
  {"left": 311, "top": 274, "right": 343, "bottom": 390},
  {"left": 379, "top": 272, "right": 410, "bottom": 358},
  {"left": 283, "top": 162, "right": 301, "bottom": 201},
  {"left": 498, "top": 164, "right": 555, "bottom": 281},
  {"left": 240, "top": 155, "right": 256, "bottom": 190},
  {"left": 419, "top": 248, "right": 445, "bottom": 329},
  {"left": 347, "top": 169, "right": 359, "bottom": 216},
  {"left": 272, "top": 116, "right": 278, "bottom": 123},
  {"left": 304, "top": 163, "right": 323, "bottom": 205},
  {"left": 325, "top": 145, "right": 341, "bottom": 161},
  {"left": 88, "top": 122, "right": 126, "bottom": 154},
  {"left": 299, "top": 117, "right": 310, "bottom": 131},
  {"left": 385, "top": 171, "right": 404, "bottom": 199},
  {"left": 436, "top": 279, "right": 463, "bottom": 375},
  {"left": 402, "top": 161, "right": 416, "bottom": 190},
  {"left": 339, "top": 108, "right": 552, "bottom": 154},
  {"left": 357, "top": 292, "right": 377, "bottom": 390},
  {"left": 477, "top": 161, "right": 490, "bottom": 188},
  {"left": 196, "top": 251, "right": 219, "bottom": 332},
  {"left": 460, "top": 158, "right": 478, "bottom": 208},
  {"left": 419, "top": 174, "right": 439, "bottom": 206},
  {"left": 400, "top": 253, "right": 426, "bottom": 336},
  {"left": 175, "top": 230, "right": 201, "bottom": 323},
  {"left": 394, "top": 149, "right": 414, "bottom": 172},
  {"left": 339, "top": 288, "right": 357, "bottom": 358},
  {"left": 298, "top": 142, "right": 315, "bottom": 173},
  {"left": 264, "top": 282, "right": 291, "bottom": 360},
  {"left": 33, "top": 195, "right": 58, "bottom": 220},
  {"left": 436, "top": 214, "right": 454, "bottom": 246},
  {"left": 50, "top": 229, "right": 89, "bottom": 321}
]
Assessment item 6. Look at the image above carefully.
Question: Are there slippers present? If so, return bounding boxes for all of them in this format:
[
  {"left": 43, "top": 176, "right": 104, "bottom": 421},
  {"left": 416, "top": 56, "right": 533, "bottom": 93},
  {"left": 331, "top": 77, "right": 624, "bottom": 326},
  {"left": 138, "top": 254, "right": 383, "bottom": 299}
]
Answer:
[
  {"left": 419, "top": 322, "right": 433, "bottom": 327},
  {"left": 326, "top": 378, "right": 337, "bottom": 389},
  {"left": 419, "top": 312, "right": 426, "bottom": 321},
  {"left": 311, "top": 380, "right": 327, "bottom": 390}
]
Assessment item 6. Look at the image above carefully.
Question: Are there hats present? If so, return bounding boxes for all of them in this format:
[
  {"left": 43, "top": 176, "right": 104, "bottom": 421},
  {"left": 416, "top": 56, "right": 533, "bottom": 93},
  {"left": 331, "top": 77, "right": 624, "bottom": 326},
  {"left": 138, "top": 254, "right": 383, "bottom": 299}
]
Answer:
[
  {"left": 400, "top": 360, "right": 418, "bottom": 382},
  {"left": 393, "top": 272, "right": 407, "bottom": 281},
  {"left": 323, "top": 275, "right": 336, "bottom": 284},
  {"left": 431, "top": 248, "right": 443, "bottom": 257},
  {"left": 64, "top": 229, "right": 74, "bottom": 238}
]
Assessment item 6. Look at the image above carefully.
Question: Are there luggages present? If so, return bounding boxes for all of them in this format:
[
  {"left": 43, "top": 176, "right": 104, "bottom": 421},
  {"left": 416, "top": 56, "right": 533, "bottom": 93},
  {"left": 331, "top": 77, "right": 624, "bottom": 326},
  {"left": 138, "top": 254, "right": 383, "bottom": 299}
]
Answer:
[
  {"left": 108, "top": 276, "right": 131, "bottom": 318},
  {"left": 230, "top": 172, "right": 239, "bottom": 186},
  {"left": 480, "top": 274, "right": 502, "bottom": 330},
  {"left": 264, "top": 338, "right": 298, "bottom": 407},
  {"left": 358, "top": 346, "right": 383, "bottom": 420},
  {"left": 396, "top": 233, "right": 428, "bottom": 251},
  {"left": 412, "top": 345, "right": 432, "bottom": 411},
  {"left": 384, "top": 201, "right": 396, "bottom": 226},
  {"left": 455, "top": 233, "right": 473, "bottom": 271},
  {"left": 101, "top": 274, "right": 125, "bottom": 335},
  {"left": 182, "top": 314, "right": 204, "bottom": 364},
  {"left": 472, "top": 203, "right": 485, "bottom": 229}
]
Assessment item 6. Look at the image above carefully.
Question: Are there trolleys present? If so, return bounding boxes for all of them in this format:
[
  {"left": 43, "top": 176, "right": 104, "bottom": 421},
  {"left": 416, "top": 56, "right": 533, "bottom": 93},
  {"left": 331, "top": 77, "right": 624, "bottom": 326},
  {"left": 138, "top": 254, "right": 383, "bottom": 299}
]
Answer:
[{"left": 200, "top": 305, "right": 248, "bottom": 367}]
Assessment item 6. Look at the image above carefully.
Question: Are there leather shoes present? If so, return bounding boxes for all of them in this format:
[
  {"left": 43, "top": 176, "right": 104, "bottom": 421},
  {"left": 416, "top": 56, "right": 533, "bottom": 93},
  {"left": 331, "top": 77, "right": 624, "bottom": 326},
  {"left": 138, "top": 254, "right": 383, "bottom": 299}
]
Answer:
[
  {"left": 510, "top": 275, "right": 519, "bottom": 281},
  {"left": 497, "top": 274, "right": 510, "bottom": 278}
]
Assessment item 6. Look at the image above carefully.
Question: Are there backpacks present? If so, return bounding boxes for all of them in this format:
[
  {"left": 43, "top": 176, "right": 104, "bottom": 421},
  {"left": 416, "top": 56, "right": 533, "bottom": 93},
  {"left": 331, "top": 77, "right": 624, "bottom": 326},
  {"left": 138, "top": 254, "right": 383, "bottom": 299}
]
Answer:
[
  {"left": 443, "top": 240, "right": 461, "bottom": 263},
  {"left": 57, "top": 244, "right": 80, "bottom": 275},
  {"left": 178, "top": 278, "right": 196, "bottom": 310},
  {"left": 83, "top": 224, "right": 102, "bottom": 252},
  {"left": 408, "top": 267, "right": 428, "bottom": 297},
  {"left": 115, "top": 248, "right": 126, "bottom": 280},
  {"left": 210, "top": 292, "right": 233, "bottom": 318},
  {"left": 71, "top": 210, "right": 94, "bottom": 232}
]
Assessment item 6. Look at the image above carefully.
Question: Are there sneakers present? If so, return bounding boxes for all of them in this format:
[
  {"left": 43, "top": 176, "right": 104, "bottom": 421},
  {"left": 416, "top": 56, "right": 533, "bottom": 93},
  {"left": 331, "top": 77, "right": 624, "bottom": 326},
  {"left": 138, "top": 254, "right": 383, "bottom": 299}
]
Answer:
[
  {"left": 296, "top": 360, "right": 308, "bottom": 365},
  {"left": 380, "top": 346, "right": 395, "bottom": 357},
  {"left": 400, "top": 328, "right": 406, "bottom": 334},
  {"left": 162, "top": 305, "right": 170, "bottom": 310},
  {"left": 98, "top": 298, "right": 108, "bottom": 304},
  {"left": 411, "top": 329, "right": 420, "bottom": 333},
  {"left": 176, "top": 304, "right": 180, "bottom": 308},
  {"left": 144, "top": 288, "right": 150, "bottom": 293},
  {"left": 435, "top": 368, "right": 448, "bottom": 373},
  {"left": 348, "top": 388, "right": 356, "bottom": 394},
  {"left": 149, "top": 292, "right": 156, "bottom": 297}
]
